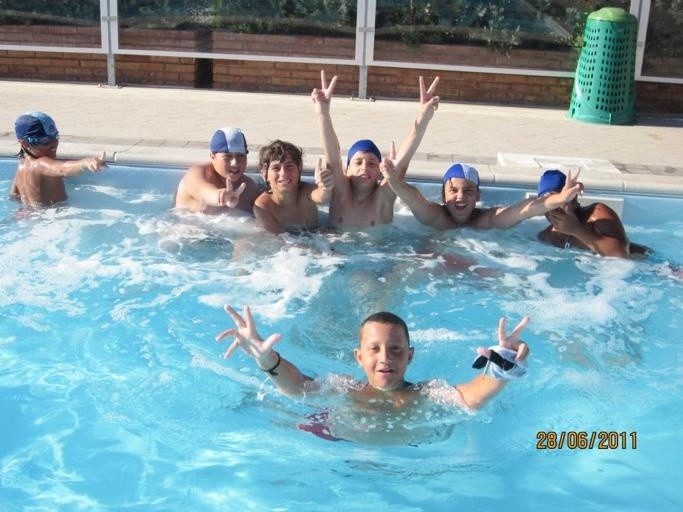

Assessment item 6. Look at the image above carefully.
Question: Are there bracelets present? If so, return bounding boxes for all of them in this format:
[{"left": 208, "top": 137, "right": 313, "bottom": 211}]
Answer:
[{"left": 79, "top": 158, "right": 86, "bottom": 173}]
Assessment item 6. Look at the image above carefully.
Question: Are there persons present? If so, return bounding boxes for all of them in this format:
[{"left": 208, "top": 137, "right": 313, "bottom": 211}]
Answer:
[
  {"left": 538, "top": 170, "right": 650, "bottom": 260},
  {"left": 380, "top": 141, "right": 584, "bottom": 231},
  {"left": 310, "top": 69, "right": 440, "bottom": 248},
  {"left": 0, "top": 110, "right": 108, "bottom": 225},
  {"left": 215, "top": 303, "right": 531, "bottom": 490},
  {"left": 176, "top": 127, "right": 261, "bottom": 216},
  {"left": 253, "top": 141, "right": 335, "bottom": 232}
]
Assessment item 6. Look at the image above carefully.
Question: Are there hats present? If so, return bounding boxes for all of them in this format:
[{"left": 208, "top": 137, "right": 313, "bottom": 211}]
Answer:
[
  {"left": 348, "top": 140, "right": 381, "bottom": 164},
  {"left": 211, "top": 129, "right": 248, "bottom": 154},
  {"left": 537, "top": 170, "right": 566, "bottom": 197},
  {"left": 15, "top": 111, "right": 57, "bottom": 139},
  {"left": 443, "top": 163, "right": 479, "bottom": 187}
]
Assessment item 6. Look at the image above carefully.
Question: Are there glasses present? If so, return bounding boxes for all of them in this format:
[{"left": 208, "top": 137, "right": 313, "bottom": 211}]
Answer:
[{"left": 23, "top": 130, "right": 59, "bottom": 144}]
